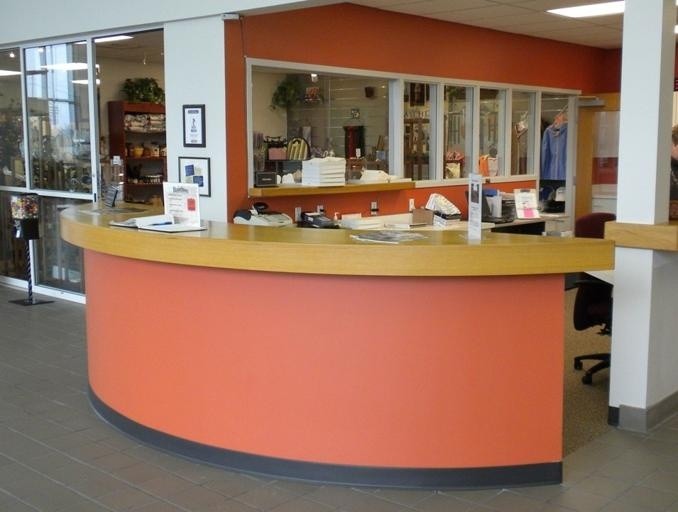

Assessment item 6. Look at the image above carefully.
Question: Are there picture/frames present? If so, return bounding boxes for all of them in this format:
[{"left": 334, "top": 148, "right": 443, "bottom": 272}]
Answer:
[
  {"left": 176, "top": 155, "right": 213, "bottom": 199},
  {"left": 181, "top": 101, "right": 206, "bottom": 150}
]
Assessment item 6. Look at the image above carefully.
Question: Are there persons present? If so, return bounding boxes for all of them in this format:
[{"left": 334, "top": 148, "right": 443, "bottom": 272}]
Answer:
[{"left": 670, "top": 124, "right": 678, "bottom": 220}]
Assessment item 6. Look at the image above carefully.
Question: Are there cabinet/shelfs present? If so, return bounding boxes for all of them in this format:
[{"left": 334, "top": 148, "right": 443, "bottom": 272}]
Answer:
[{"left": 105, "top": 97, "right": 168, "bottom": 206}]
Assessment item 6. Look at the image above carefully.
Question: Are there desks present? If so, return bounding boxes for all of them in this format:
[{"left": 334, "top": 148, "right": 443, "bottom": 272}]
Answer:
[{"left": 430, "top": 212, "right": 570, "bottom": 238}]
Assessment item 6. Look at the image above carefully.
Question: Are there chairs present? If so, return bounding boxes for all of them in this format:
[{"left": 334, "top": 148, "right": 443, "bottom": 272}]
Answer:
[{"left": 567, "top": 209, "right": 613, "bottom": 388}]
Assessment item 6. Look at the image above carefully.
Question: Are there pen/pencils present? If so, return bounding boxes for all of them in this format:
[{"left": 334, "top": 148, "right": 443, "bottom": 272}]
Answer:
[{"left": 152, "top": 222, "right": 172, "bottom": 226}]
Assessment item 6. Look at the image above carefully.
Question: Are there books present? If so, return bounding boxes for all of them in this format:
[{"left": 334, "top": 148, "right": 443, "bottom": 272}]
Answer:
[
  {"left": 109, "top": 213, "right": 207, "bottom": 234},
  {"left": 302, "top": 156, "right": 346, "bottom": 187}
]
